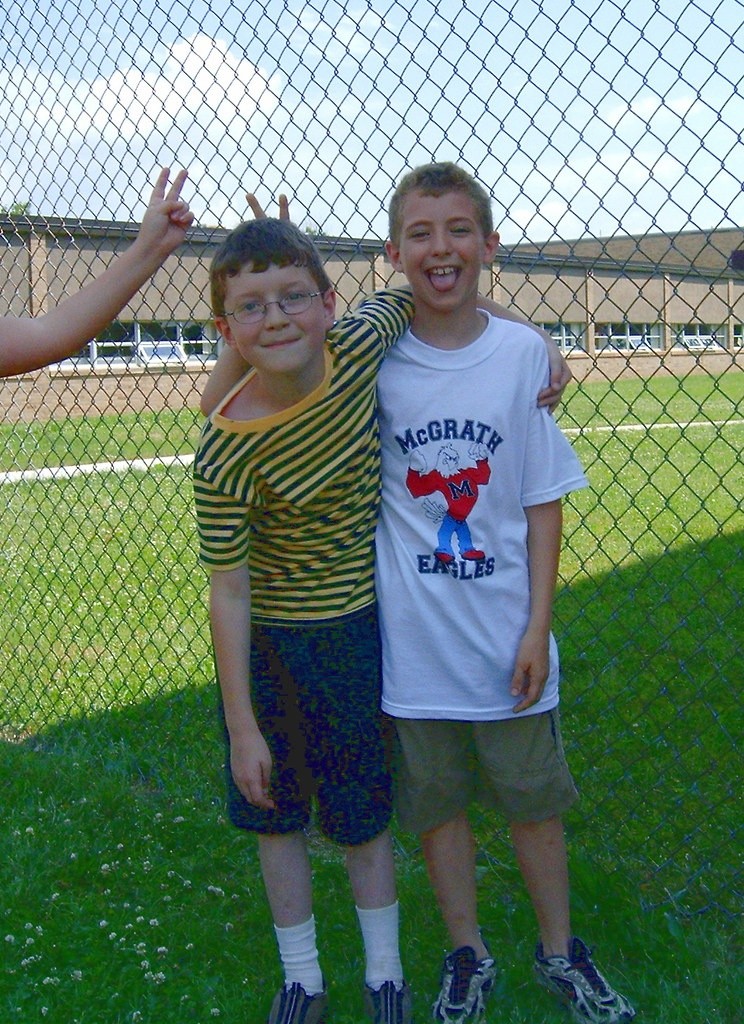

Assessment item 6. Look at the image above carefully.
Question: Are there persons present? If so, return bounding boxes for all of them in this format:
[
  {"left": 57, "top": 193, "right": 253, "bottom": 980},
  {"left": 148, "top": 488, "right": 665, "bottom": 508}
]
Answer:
[
  {"left": 0, "top": 167, "right": 195, "bottom": 378},
  {"left": 191, "top": 217, "right": 573, "bottom": 1024},
  {"left": 200, "top": 161, "right": 637, "bottom": 1024}
]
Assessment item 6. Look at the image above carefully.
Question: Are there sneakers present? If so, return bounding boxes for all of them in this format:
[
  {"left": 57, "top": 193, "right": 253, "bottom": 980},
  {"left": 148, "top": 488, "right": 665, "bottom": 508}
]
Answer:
[
  {"left": 363, "top": 980, "right": 413, "bottom": 1024},
  {"left": 431, "top": 932, "right": 496, "bottom": 1024},
  {"left": 533, "top": 937, "right": 637, "bottom": 1023},
  {"left": 265, "top": 974, "right": 327, "bottom": 1024}
]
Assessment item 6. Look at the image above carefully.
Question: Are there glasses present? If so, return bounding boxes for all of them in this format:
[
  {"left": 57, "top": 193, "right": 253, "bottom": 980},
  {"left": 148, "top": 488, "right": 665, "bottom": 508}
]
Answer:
[{"left": 222, "top": 291, "right": 324, "bottom": 324}]
